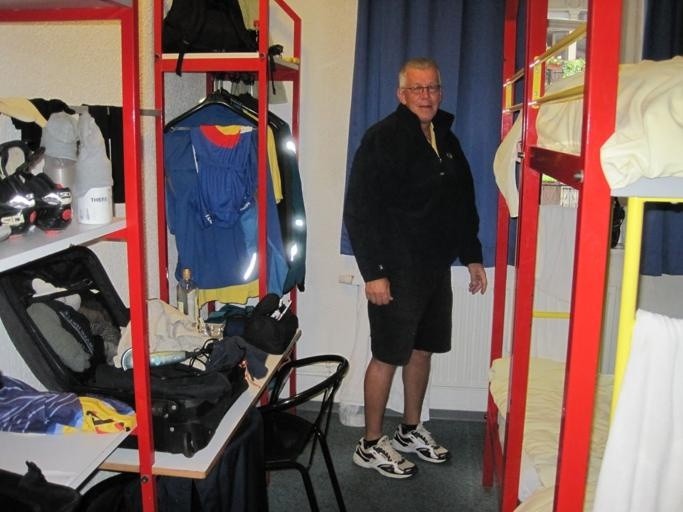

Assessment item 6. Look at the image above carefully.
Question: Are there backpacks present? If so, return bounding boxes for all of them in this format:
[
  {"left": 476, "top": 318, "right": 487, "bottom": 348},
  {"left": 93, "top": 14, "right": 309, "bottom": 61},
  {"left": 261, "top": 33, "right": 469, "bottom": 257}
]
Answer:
[{"left": 163, "top": 0, "right": 282, "bottom": 54}]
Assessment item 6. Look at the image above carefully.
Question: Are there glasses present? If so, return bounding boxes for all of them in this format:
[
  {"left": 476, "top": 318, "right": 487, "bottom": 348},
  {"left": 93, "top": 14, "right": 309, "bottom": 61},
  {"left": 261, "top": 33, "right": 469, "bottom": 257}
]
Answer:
[{"left": 400, "top": 84, "right": 442, "bottom": 93}]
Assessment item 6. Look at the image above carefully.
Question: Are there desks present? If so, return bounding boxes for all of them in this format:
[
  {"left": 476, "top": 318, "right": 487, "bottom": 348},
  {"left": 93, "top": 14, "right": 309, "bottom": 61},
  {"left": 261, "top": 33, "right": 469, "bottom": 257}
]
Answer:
[{"left": 99, "top": 324, "right": 302, "bottom": 512}]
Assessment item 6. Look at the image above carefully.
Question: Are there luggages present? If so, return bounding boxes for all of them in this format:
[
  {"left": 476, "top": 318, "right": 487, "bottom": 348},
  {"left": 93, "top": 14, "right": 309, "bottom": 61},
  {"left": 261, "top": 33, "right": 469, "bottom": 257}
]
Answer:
[{"left": 0, "top": 243, "right": 248, "bottom": 458}]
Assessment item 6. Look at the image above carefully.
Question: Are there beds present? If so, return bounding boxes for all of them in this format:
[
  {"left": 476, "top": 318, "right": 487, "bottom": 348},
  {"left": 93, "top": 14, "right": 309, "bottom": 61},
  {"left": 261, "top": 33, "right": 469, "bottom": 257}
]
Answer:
[{"left": 483, "top": 58, "right": 682, "bottom": 511}]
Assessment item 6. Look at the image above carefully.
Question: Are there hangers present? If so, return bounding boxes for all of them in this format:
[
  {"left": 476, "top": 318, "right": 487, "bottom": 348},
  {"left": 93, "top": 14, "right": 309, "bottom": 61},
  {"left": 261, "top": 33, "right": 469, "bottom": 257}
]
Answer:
[{"left": 163, "top": 74, "right": 281, "bottom": 135}]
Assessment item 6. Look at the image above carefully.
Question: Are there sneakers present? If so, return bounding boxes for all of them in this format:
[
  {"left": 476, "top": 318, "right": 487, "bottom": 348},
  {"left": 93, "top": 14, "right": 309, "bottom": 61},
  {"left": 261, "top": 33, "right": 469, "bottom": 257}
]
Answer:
[
  {"left": 351, "top": 433, "right": 419, "bottom": 479},
  {"left": 391, "top": 423, "right": 450, "bottom": 464}
]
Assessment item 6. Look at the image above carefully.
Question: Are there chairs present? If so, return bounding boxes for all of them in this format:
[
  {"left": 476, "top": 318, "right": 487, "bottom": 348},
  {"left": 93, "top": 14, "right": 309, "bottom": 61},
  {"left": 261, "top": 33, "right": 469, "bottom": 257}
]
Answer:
[{"left": 253, "top": 355, "right": 349, "bottom": 512}]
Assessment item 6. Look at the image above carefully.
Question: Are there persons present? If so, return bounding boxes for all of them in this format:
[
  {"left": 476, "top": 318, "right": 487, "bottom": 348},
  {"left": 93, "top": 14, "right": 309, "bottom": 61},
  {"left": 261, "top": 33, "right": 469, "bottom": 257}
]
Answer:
[{"left": 342, "top": 57, "right": 489, "bottom": 480}]
